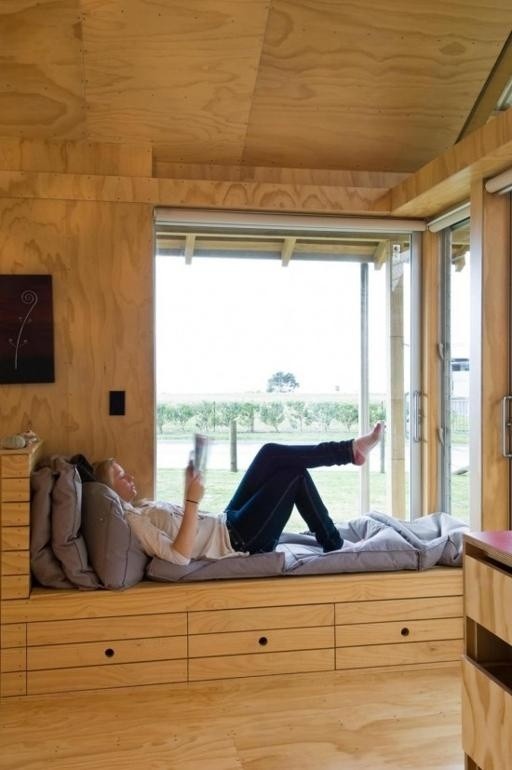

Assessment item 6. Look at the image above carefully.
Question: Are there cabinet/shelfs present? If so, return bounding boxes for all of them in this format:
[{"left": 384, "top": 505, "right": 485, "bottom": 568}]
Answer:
[{"left": 462, "top": 532, "right": 512, "bottom": 768}]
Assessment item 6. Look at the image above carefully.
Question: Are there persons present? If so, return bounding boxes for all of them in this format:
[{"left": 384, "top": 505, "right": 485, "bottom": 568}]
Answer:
[{"left": 91, "top": 421, "right": 386, "bottom": 567}]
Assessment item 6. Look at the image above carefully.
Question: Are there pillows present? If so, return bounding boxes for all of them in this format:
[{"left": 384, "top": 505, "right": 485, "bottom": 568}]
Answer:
[{"left": 30, "top": 458, "right": 149, "bottom": 590}]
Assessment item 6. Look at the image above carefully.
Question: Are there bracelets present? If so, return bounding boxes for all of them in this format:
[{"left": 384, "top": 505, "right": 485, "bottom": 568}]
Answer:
[{"left": 186, "top": 499, "right": 199, "bottom": 505}]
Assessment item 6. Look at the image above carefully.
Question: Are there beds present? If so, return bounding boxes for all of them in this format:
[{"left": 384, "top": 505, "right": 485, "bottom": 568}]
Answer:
[{"left": 2, "top": 441, "right": 461, "bottom": 692}]
